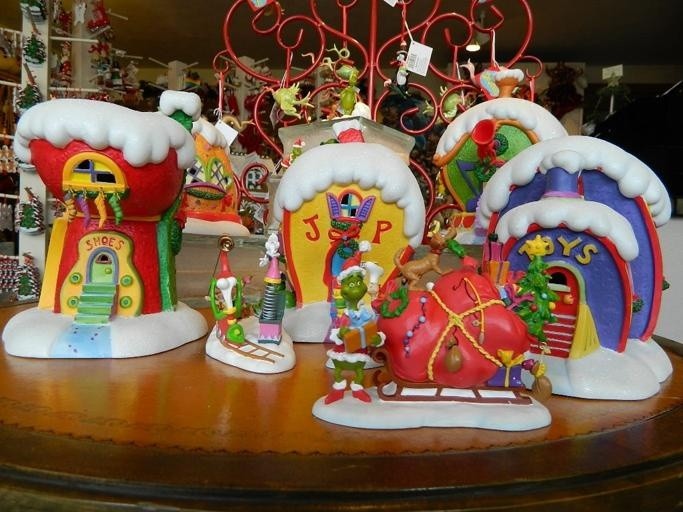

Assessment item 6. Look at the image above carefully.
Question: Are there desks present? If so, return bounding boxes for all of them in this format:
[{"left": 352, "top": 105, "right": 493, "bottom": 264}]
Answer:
[{"left": 1, "top": 290, "right": 683, "bottom": 511}]
